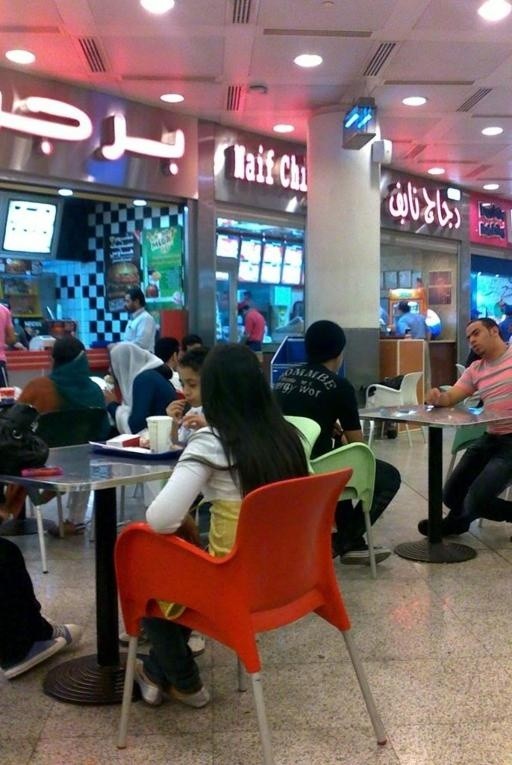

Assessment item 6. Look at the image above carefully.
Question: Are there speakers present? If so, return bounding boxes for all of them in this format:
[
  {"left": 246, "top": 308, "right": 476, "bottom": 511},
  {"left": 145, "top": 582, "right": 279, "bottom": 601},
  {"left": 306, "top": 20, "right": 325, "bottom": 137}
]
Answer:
[{"left": 372, "top": 139, "right": 393, "bottom": 164}]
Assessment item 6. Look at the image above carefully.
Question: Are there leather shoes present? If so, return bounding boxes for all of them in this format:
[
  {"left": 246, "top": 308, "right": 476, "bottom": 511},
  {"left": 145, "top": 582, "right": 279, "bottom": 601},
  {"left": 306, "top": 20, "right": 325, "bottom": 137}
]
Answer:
[{"left": 135, "top": 663, "right": 210, "bottom": 709}]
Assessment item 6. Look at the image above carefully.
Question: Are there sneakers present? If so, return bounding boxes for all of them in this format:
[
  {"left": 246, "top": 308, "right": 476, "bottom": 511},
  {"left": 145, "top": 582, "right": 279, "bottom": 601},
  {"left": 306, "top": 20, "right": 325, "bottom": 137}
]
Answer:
[
  {"left": 341, "top": 546, "right": 391, "bottom": 565},
  {"left": 188, "top": 630, "right": 205, "bottom": 657},
  {"left": 418, "top": 519, "right": 469, "bottom": 535},
  {"left": 119, "top": 631, "right": 146, "bottom": 646},
  {"left": 1, "top": 620, "right": 81, "bottom": 680}
]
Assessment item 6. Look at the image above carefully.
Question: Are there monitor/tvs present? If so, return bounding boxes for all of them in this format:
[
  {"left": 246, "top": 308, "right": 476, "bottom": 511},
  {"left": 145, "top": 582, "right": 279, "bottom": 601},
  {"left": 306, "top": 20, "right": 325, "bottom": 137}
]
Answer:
[{"left": 0, "top": 189, "right": 65, "bottom": 261}]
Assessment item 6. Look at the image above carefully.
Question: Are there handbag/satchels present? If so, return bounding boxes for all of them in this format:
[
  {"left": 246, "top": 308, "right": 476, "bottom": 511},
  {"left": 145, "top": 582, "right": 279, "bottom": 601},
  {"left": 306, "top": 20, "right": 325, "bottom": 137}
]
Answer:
[{"left": 0, "top": 403, "right": 48, "bottom": 475}]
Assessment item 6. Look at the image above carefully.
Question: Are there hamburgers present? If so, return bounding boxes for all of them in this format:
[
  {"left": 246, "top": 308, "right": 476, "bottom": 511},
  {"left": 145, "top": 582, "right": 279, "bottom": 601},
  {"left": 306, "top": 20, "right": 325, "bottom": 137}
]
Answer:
[
  {"left": 105, "top": 261, "right": 142, "bottom": 299},
  {"left": 5, "top": 258, "right": 27, "bottom": 275}
]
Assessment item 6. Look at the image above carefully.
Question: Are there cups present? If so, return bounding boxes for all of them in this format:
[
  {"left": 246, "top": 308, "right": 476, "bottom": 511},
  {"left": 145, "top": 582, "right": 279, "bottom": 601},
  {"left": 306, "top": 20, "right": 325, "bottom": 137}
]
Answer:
[
  {"left": 145, "top": 414, "right": 173, "bottom": 455},
  {"left": 0, "top": 386, "right": 15, "bottom": 404}
]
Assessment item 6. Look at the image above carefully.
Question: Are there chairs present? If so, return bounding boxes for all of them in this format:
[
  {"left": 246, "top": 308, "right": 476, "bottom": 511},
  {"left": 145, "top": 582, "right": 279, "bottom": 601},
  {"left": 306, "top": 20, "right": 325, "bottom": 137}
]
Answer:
[
  {"left": 359, "top": 371, "right": 427, "bottom": 447},
  {"left": 448, "top": 425, "right": 512, "bottom": 526},
  {"left": 32, "top": 407, "right": 113, "bottom": 450},
  {"left": 283, "top": 414, "right": 377, "bottom": 578},
  {"left": 114, "top": 469, "right": 389, "bottom": 765}
]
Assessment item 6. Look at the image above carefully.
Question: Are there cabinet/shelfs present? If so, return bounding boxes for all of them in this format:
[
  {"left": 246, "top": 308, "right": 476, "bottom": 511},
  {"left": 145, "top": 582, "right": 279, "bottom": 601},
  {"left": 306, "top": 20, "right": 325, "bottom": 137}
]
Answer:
[
  {"left": 0, "top": 254, "right": 44, "bottom": 337},
  {"left": 378, "top": 339, "right": 425, "bottom": 409}
]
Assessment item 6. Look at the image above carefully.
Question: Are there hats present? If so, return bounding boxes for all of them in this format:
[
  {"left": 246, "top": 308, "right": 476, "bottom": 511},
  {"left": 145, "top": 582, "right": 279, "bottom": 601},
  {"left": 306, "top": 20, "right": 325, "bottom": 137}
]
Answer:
[{"left": 305, "top": 320, "right": 346, "bottom": 362}]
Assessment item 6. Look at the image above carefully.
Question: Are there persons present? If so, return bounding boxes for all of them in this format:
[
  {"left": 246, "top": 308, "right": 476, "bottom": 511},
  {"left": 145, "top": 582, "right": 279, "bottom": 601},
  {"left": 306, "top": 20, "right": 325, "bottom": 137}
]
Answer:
[
  {"left": 0, "top": 281, "right": 16, "bottom": 389},
  {"left": 0, "top": 388, "right": 82, "bottom": 679},
  {"left": 133, "top": 341, "right": 316, "bottom": 711},
  {"left": 497, "top": 303, "right": 512, "bottom": 341},
  {"left": 102, "top": 334, "right": 213, "bottom": 448},
  {"left": 15, "top": 337, "right": 105, "bottom": 535},
  {"left": 273, "top": 318, "right": 401, "bottom": 565},
  {"left": 237, "top": 303, "right": 266, "bottom": 351},
  {"left": 392, "top": 301, "right": 434, "bottom": 399},
  {"left": 244, "top": 292, "right": 258, "bottom": 310},
  {"left": 416, "top": 317, "right": 512, "bottom": 541},
  {"left": 123, "top": 287, "right": 155, "bottom": 355}
]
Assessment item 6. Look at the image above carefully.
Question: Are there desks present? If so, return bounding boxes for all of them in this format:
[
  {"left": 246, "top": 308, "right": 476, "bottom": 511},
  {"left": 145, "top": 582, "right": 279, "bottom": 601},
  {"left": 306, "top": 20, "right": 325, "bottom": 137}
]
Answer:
[
  {"left": 2, "top": 443, "right": 182, "bottom": 707},
  {"left": 358, "top": 404, "right": 512, "bottom": 564}
]
